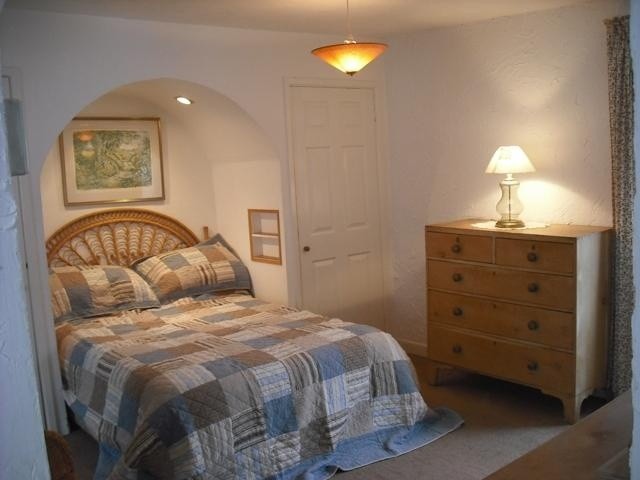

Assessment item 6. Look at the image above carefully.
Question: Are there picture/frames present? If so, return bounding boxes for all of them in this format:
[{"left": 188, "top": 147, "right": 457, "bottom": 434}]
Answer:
[{"left": 58, "top": 117, "right": 165, "bottom": 208}]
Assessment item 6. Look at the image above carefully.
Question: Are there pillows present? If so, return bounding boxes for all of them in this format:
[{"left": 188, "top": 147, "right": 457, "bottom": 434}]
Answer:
[{"left": 46, "top": 234, "right": 253, "bottom": 331}]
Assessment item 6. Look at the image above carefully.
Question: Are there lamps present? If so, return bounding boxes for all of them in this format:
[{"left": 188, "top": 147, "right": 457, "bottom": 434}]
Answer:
[
  {"left": 485, "top": 145, "right": 537, "bottom": 229},
  {"left": 310, "top": 0, "right": 389, "bottom": 79}
]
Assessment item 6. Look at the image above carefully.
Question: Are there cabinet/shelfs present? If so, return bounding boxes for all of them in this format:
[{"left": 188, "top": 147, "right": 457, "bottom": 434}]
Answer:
[
  {"left": 424, "top": 217, "right": 615, "bottom": 426},
  {"left": 248, "top": 209, "right": 281, "bottom": 266}
]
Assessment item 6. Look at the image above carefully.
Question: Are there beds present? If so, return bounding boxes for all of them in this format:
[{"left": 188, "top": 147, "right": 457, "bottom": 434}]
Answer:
[{"left": 40, "top": 208, "right": 430, "bottom": 477}]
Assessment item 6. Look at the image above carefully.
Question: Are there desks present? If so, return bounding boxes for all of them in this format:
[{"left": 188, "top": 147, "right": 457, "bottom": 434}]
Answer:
[{"left": 483, "top": 389, "right": 631, "bottom": 479}]
